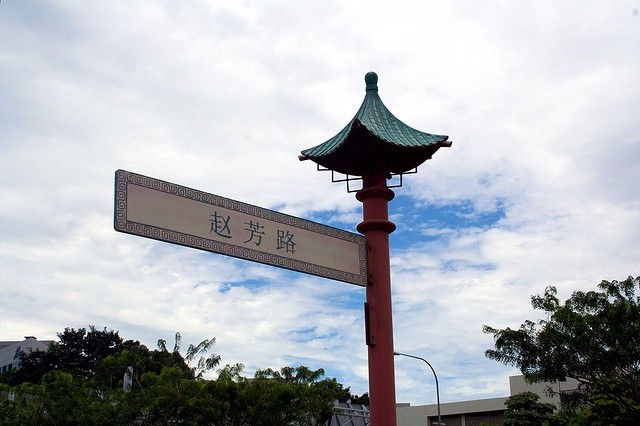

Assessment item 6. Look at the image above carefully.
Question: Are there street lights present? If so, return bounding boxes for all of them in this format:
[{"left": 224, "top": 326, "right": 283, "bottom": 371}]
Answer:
[{"left": 393, "top": 352, "right": 441, "bottom": 426}]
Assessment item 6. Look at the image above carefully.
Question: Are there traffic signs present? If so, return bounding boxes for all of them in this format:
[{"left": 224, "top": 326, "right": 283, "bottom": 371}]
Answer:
[{"left": 114, "top": 170, "right": 367, "bottom": 286}]
